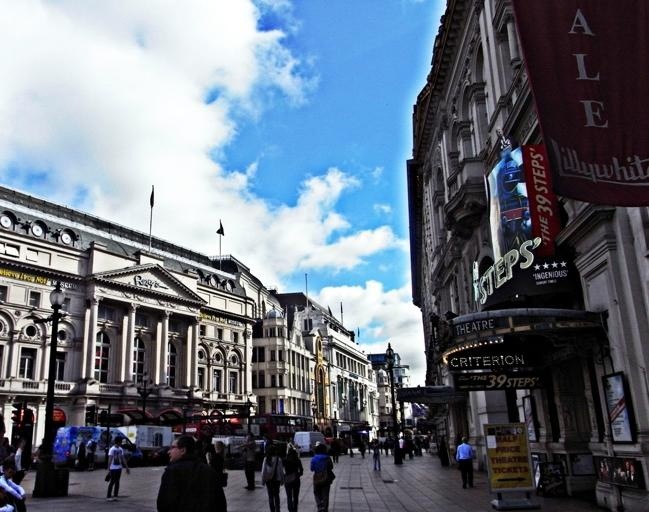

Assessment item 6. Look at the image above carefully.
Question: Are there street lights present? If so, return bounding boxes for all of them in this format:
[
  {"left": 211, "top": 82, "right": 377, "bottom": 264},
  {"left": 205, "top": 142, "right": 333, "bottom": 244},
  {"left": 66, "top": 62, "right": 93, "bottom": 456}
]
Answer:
[
  {"left": 27, "top": 275, "right": 70, "bottom": 496},
  {"left": 311, "top": 399, "right": 317, "bottom": 431},
  {"left": 385, "top": 342, "right": 403, "bottom": 464},
  {"left": 245, "top": 395, "right": 254, "bottom": 434},
  {"left": 396, "top": 373, "right": 406, "bottom": 431},
  {"left": 138, "top": 372, "right": 154, "bottom": 422}
]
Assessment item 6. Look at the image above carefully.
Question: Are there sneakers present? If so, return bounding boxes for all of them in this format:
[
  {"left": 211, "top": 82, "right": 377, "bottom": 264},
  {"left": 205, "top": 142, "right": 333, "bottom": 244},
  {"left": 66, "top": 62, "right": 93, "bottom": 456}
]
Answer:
[{"left": 106, "top": 497, "right": 120, "bottom": 502}]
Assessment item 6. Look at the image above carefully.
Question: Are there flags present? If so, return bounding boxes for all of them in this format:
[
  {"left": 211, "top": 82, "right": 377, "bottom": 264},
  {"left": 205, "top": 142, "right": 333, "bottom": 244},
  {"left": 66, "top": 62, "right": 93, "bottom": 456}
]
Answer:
[
  {"left": 150, "top": 185, "right": 154, "bottom": 207},
  {"left": 216, "top": 221, "right": 224, "bottom": 236}
]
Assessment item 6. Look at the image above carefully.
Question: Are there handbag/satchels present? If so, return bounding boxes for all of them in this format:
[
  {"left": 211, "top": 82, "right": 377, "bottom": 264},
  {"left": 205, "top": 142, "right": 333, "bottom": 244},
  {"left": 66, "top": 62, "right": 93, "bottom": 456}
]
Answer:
[
  {"left": 261, "top": 470, "right": 277, "bottom": 483},
  {"left": 222, "top": 469, "right": 229, "bottom": 487},
  {"left": 313, "top": 470, "right": 335, "bottom": 484},
  {"left": 283, "top": 471, "right": 301, "bottom": 485}
]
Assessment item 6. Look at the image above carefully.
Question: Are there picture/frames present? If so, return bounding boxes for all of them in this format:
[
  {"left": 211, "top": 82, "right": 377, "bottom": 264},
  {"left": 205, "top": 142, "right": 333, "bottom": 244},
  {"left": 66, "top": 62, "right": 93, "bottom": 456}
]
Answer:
[
  {"left": 521, "top": 394, "right": 541, "bottom": 443},
  {"left": 601, "top": 370, "right": 639, "bottom": 444}
]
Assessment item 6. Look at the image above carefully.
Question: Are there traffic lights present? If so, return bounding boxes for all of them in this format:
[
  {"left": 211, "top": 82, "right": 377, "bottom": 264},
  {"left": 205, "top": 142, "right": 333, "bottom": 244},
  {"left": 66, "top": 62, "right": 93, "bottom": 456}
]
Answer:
[{"left": 10, "top": 403, "right": 21, "bottom": 424}]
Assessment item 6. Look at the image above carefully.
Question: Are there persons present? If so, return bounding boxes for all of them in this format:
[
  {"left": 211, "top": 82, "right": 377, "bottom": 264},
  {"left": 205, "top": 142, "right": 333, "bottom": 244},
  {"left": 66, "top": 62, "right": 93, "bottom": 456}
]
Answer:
[{"left": 457, "top": 437, "right": 475, "bottom": 488}]
